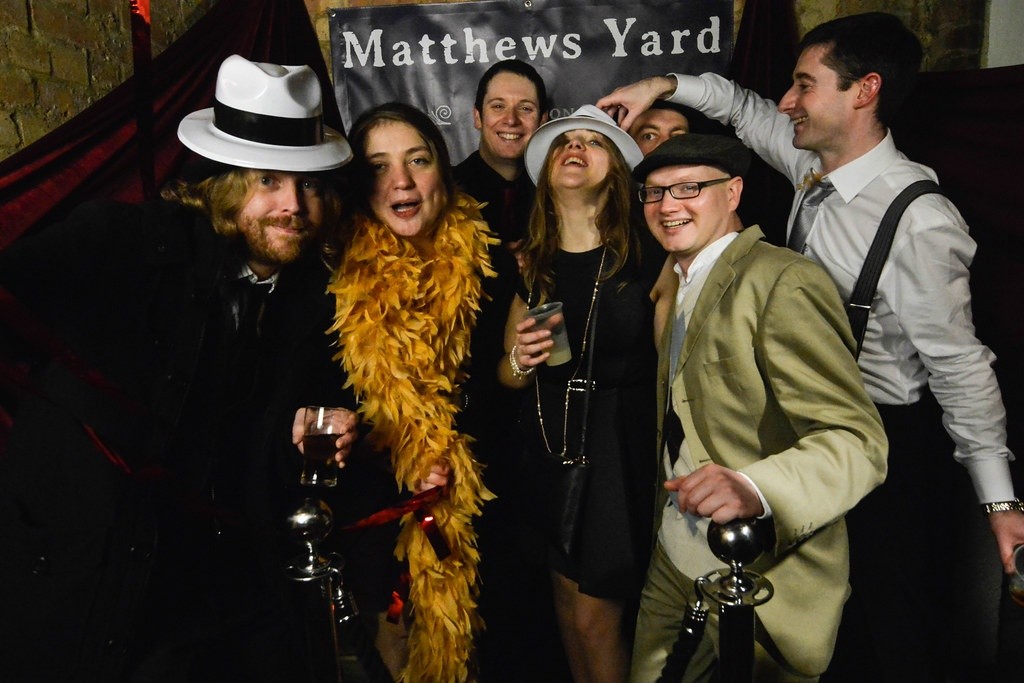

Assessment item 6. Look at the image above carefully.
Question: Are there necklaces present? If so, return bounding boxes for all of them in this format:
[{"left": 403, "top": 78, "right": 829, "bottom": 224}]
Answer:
[{"left": 526, "top": 236, "right": 612, "bottom": 460}]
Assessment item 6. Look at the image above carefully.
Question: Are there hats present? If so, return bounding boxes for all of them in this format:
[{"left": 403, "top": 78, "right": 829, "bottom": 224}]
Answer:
[
  {"left": 523, "top": 102, "right": 643, "bottom": 187},
  {"left": 633, "top": 135, "right": 745, "bottom": 183},
  {"left": 174, "top": 55, "right": 351, "bottom": 173}
]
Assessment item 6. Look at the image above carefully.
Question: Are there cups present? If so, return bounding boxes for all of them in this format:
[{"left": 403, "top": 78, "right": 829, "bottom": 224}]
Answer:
[
  {"left": 523, "top": 301, "right": 572, "bottom": 366},
  {"left": 1009, "top": 545, "right": 1024, "bottom": 606},
  {"left": 300, "top": 406, "right": 348, "bottom": 488}
]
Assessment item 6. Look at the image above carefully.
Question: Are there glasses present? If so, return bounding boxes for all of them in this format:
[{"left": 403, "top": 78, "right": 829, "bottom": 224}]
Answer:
[{"left": 638, "top": 176, "right": 733, "bottom": 204}]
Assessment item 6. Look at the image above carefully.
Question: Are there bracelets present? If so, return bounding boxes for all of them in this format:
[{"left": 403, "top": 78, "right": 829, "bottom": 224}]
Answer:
[{"left": 507, "top": 344, "right": 538, "bottom": 383}]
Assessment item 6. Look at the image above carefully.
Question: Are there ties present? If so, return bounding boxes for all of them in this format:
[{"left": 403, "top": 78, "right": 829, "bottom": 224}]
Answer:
[{"left": 786, "top": 185, "right": 836, "bottom": 253}]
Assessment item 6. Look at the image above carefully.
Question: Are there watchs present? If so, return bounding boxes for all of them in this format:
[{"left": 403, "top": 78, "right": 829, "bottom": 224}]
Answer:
[{"left": 980, "top": 495, "right": 1024, "bottom": 521}]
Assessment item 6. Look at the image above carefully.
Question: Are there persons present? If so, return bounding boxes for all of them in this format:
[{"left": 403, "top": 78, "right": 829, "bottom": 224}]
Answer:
[
  {"left": 444, "top": 56, "right": 562, "bottom": 679},
  {"left": 311, "top": 100, "right": 515, "bottom": 681},
  {"left": 17, "top": 94, "right": 358, "bottom": 674},
  {"left": 488, "top": 108, "right": 671, "bottom": 683},
  {"left": 587, "top": 4, "right": 1024, "bottom": 683},
  {"left": 622, "top": 100, "right": 692, "bottom": 165},
  {"left": 627, "top": 129, "right": 890, "bottom": 683}
]
